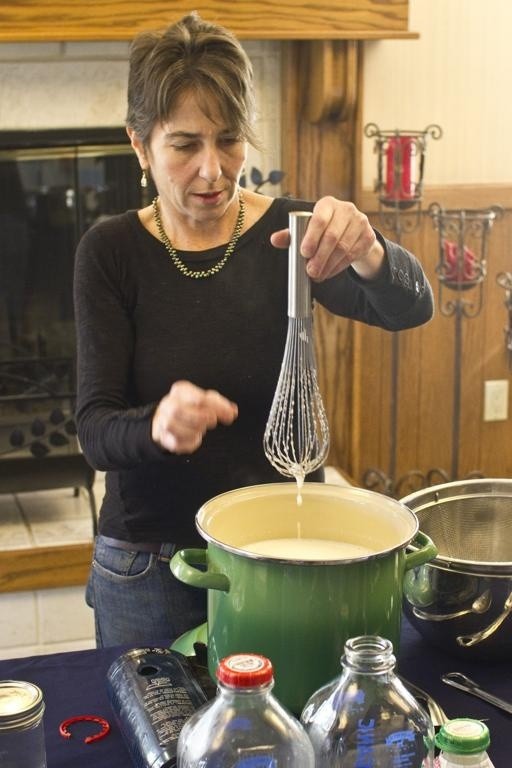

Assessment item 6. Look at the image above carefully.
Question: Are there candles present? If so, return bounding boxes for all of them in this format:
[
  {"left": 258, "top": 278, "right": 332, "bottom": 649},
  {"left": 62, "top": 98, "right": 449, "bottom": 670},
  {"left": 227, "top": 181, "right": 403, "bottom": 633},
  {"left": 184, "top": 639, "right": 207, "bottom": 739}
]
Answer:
[
  {"left": 445, "top": 242, "right": 474, "bottom": 281},
  {"left": 385, "top": 136, "right": 415, "bottom": 201}
]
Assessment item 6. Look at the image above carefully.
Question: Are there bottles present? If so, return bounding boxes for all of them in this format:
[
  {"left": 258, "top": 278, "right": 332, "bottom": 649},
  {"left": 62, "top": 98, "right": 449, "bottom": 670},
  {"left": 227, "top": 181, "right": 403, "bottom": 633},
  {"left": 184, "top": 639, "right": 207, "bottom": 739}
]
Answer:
[
  {"left": 429, "top": 719, "right": 497, "bottom": 768},
  {"left": 300, "top": 635, "right": 435, "bottom": 766},
  {"left": 0, "top": 681, "right": 46, "bottom": 768},
  {"left": 177, "top": 652, "right": 316, "bottom": 766}
]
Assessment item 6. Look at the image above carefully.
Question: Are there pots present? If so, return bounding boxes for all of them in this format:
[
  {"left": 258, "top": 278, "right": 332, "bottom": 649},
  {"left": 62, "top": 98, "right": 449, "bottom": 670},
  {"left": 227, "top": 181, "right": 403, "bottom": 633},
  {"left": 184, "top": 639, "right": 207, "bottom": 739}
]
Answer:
[
  {"left": 170, "top": 479, "right": 440, "bottom": 715},
  {"left": 373, "top": 478, "right": 512, "bottom": 662}
]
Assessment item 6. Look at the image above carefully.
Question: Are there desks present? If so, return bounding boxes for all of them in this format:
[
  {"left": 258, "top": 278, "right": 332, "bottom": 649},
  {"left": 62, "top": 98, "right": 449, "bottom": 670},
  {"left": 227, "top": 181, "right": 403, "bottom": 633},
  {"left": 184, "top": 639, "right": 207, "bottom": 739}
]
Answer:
[{"left": 0, "top": 612, "right": 511, "bottom": 767}]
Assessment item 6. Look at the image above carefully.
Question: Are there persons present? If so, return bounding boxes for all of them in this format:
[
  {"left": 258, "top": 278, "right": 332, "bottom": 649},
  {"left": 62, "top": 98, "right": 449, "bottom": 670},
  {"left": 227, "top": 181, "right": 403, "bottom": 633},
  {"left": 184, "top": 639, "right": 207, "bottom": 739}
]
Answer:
[{"left": 74, "top": 11, "right": 435, "bottom": 651}]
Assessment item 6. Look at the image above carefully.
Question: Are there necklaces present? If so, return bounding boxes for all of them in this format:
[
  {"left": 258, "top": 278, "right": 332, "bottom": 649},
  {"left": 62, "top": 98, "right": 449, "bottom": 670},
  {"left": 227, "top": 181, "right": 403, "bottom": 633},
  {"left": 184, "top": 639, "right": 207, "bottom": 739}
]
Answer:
[{"left": 151, "top": 186, "right": 244, "bottom": 278}]
